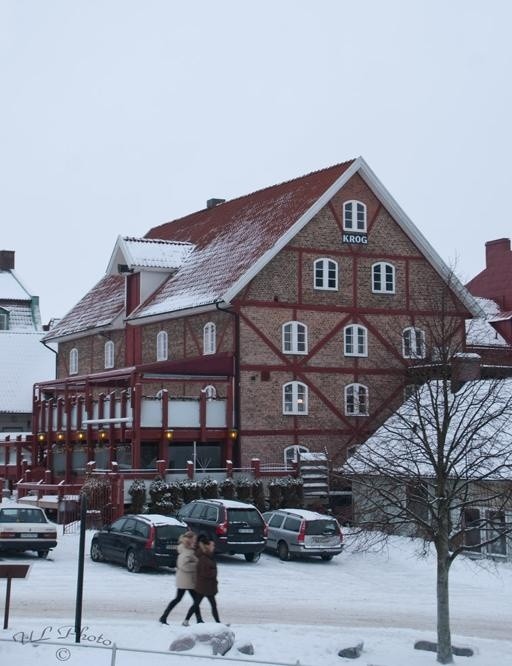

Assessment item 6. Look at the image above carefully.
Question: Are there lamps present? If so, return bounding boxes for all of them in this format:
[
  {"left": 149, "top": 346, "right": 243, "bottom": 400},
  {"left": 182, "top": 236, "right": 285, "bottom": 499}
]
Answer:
[
  {"left": 56, "top": 431, "right": 63, "bottom": 441},
  {"left": 165, "top": 429, "right": 174, "bottom": 440},
  {"left": 98, "top": 430, "right": 106, "bottom": 441},
  {"left": 77, "top": 431, "right": 85, "bottom": 441},
  {"left": 229, "top": 429, "right": 239, "bottom": 440},
  {"left": 37, "top": 432, "right": 46, "bottom": 443}
]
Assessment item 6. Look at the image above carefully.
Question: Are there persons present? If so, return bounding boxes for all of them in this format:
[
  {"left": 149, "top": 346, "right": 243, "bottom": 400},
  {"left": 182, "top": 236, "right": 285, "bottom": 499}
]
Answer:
[
  {"left": 179, "top": 536, "right": 232, "bottom": 628},
  {"left": 157, "top": 530, "right": 207, "bottom": 625}
]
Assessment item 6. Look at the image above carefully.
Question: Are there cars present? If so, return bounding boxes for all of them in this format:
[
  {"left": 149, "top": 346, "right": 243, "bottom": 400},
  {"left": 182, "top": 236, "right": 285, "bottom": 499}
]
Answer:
[
  {"left": 89, "top": 497, "right": 345, "bottom": 573},
  {"left": 0, "top": 504, "right": 57, "bottom": 557}
]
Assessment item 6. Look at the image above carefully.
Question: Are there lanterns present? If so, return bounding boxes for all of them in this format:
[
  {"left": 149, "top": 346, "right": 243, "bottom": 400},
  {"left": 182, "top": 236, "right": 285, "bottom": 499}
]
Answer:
[
  {"left": 37, "top": 433, "right": 45, "bottom": 441},
  {"left": 228, "top": 428, "right": 238, "bottom": 441},
  {"left": 163, "top": 429, "right": 174, "bottom": 443},
  {"left": 55, "top": 431, "right": 65, "bottom": 442},
  {"left": 98, "top": 430, "right": 108, "bottom": 442},
  {"left": 76, "top": 431, "right": 86, "bottom": 442}
]
